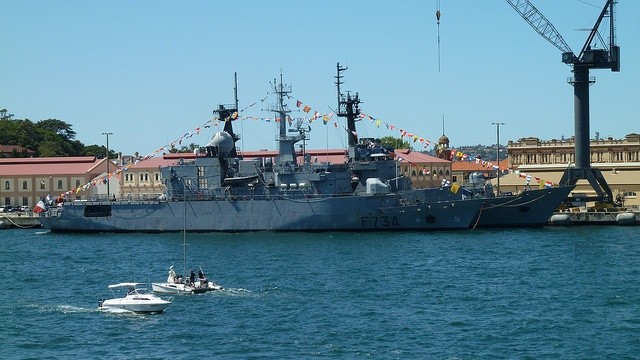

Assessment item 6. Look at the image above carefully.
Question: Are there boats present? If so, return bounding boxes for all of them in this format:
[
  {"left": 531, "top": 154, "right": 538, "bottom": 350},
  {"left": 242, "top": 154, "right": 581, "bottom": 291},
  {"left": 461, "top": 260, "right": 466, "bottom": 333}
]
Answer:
[
  {"left": 97, "top": 282, "right": 170, "bottom": 313},
  {"left": 152, "top": 279, "right": 224, "bottom": 294}
]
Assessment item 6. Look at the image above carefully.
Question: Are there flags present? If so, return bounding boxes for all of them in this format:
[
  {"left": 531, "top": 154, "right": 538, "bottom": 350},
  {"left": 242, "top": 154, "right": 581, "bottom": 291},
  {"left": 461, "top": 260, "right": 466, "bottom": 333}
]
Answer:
[{"left": 32, "top": 194, "right": 48, "bottom": 213}]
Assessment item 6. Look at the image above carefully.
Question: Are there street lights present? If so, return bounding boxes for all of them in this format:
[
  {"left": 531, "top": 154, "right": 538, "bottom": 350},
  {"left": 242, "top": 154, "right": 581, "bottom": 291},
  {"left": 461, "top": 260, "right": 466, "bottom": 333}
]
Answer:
[
  {"left": 493, "top": 123, "right": 504, "bottom": 196},
  {"left": 102, "top": 133, "right": 113, "bottom": 200}
]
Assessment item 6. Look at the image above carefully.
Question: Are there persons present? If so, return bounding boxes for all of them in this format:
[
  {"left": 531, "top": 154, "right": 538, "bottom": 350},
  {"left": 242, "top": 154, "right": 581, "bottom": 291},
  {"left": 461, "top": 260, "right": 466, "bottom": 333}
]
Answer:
[
  {"left": 127, "top": 286, "right": 135, "bottom": 295},
  {"left": 197, "top": 270, "right": 202, "bottom": 278},
  {"left": 177, "top": 273, "right": 182, "bottom": 280},
  {"left": 190, "top": 270, "right": 196, "bottom": 284}
]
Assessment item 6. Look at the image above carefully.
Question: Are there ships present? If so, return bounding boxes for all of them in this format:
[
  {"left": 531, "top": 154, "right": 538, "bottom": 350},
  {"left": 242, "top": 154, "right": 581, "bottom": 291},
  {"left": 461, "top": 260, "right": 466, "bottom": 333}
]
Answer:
[
  {"left": 333, "top": 63, "right": 575, "bottom": 226},
  {"left": 36, "top": 69, "right": 484, "bottom": 232}
]
